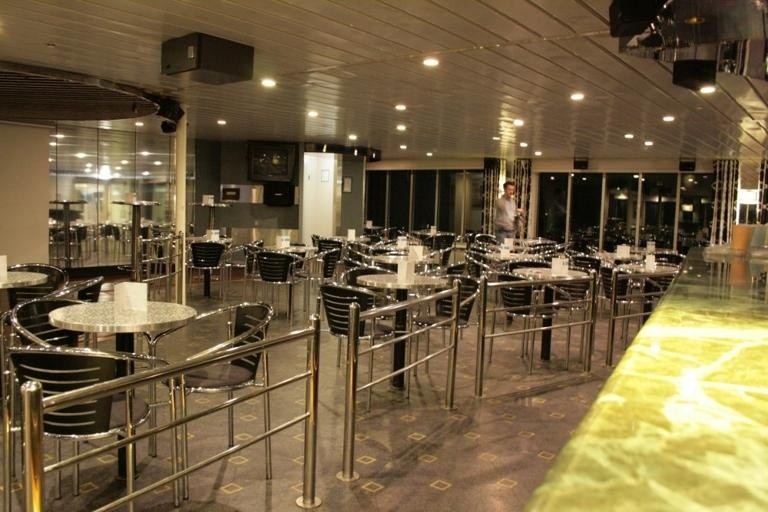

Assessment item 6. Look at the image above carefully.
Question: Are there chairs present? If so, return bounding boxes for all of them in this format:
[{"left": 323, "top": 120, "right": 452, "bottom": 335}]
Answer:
[
  {"left": 1, "top": 345, "right": 182, "bottom": 512},
  {"left": 468, "top": 233, "right": 558, "bottom": 263},
  {"left": 184, "top": 241, "right": 227, "bottom": 302},
  {"left": 464, "top": 250, "right": 497, "bottom": 278},
  {"left": 8, "top": 264, "right": 65, "bottom": 309},
  {"left": 362, "top": 227, "right": 439, "bottom": 256},
  {"left": 347, "top": 242, "right": 373, "bottom": 266},
  {"left": 1, "top": 276, "right": 104, "bottom": 361},
  {"left": 341, "top": 266, "right": 394, "bottom": 283},
  {"left": 320, "top": 281, "right": 414, "bottom": 411},
  {"left": 319, "top": 236, "right": 347, "bottom": 259},
  {"left": 11, "top": 298, "right": 98, "bottom": 348},
  {"left": 488, "top": 271, "right": 537, "bottom": 373},
  {"left": 424, "top": 233, "right": 456, "bottom": 269},
  {"left": 568, "top": 255, "right": 600, "bottom": 289},
  {"left": 644, "top": 251, "right": 685, "bottom": 292},
  {"left": 288, "top": 248, "right": 339, "bottom": 324},
  {"left": 413, "top": 274, "right": 481, "bottom": 377},
  {"left": 243, "top": 244, "right": 265, "bottom": 302},
  {"left": 343, "top": 254, "right": 364, "bottom": 271},
  {"left": 219, "top": 239, "right": 263, "bottom": 302},
  {"left": 253, "top": 249, "right": 308, "bottom": 326},
  {"left": 152, "top": 301, "right": 275, "bottom": 508},
  {"left": 595, "top": 265, "right": 633, "bottom": 339}
]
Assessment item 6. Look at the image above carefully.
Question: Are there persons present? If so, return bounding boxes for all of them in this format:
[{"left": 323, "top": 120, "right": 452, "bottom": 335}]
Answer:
[{"left": 495, "top": 182, "right": 524, "bottom": 244}]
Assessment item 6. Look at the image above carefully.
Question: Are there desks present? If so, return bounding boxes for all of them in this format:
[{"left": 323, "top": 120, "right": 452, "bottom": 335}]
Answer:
[
  {"left": 357, "top": 275, "right": 448, "bottom": 391},
  {"left": 512, "top": 268, "right": 588, "bottom": 360},
  {"left": 526, "top": 246, "right": 768, "bottom": 512},
  {"left": 49, "top": 301, "right": 198, "bottom": 458},
  {"left": 623, "top": 263, "right": 680, "bottom": 324},
  {"left": 1, "top": 271, "right": 48, "bottom": 309}
]
